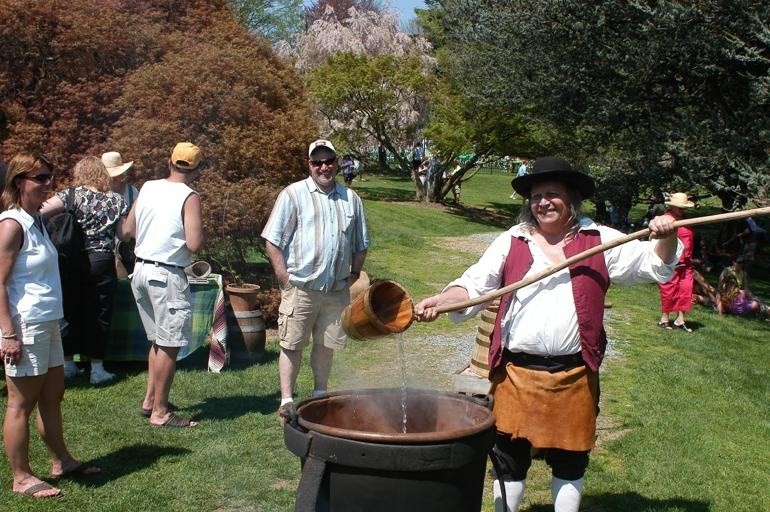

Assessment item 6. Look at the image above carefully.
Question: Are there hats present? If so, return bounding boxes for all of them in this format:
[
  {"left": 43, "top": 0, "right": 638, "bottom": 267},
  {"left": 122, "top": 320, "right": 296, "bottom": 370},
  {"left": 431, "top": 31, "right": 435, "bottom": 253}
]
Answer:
[
  {"left": 100, "top": 151, "right": 134, "bottom": 178},
  {"left": 664, "top": 192, "right": 696, "bottom": 209},
  {"left": 308, "top": 139, "right": 337, "bottom": 158},
  {"left": 511, "top": 156, "right": 596, "bottom": 200},
  {"left": 171, "top": 141, "right": 201, "bottom": 170}
]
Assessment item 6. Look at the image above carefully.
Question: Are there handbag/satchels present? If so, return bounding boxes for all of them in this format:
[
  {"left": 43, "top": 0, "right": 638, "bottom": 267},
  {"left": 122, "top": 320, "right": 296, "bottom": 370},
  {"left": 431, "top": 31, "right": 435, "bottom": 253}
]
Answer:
[{"left": 45, "top": 188, "right": 91, "bottom": 274}]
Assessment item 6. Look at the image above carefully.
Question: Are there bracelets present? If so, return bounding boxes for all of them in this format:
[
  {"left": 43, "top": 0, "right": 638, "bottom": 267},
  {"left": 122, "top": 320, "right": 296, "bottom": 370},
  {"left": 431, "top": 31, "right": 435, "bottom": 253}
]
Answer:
[
  {"left": 2, "top": 333, "right": 18, "bottom": 339},
  {"left": 349, "top": 270, "right": 360, "bottom": 279}
]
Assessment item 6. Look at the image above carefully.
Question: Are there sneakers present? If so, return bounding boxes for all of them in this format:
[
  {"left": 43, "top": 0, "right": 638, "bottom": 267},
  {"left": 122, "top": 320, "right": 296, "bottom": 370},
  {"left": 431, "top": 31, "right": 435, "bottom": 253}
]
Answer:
[{"left": 279, "top": 402, "right": 293, "bottom": 427}]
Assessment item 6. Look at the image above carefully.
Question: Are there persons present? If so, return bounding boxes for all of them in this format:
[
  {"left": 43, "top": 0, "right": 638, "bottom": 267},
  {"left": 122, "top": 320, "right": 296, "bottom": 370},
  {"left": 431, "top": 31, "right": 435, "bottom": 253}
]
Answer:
[
  {"left": 406, "top": 141, "right": 464, "bottom": 208},
  {"left": 116, "top": 140, "right": 205, "bottom": 429},
  {"left": 35, "top": 156, "right": 133, "bottom": 391},
  {"left": 351, "top": 160, "right": 367, "bottom": 182},
  {"left": 97, "top": 152, "right": 141, "bottom": 277},
  {"left": 409, "top": 153, "right": 679, "bottom": 512},
  {"left": 509, "top": 160, "right": 530, "bottom": 201},
  {"left": 648, "top": 189, "right": 697, "bottom": 335},
  {"left": 611, "top": 187, "right": 770, "bottom": 325},
  {"left": 259, "top": 136, "right": 372, "bottom": 421},
  {"left": 340, "top": 154, "right": 355, "bottom": 188},
  {"left": 1, "top": 147, "right": 101, "bottom": 502}
]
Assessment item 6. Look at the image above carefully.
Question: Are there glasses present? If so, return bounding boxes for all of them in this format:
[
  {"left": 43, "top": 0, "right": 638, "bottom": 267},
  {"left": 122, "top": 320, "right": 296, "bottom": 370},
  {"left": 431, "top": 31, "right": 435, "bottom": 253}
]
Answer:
[
  {"left": 310, "top": 156, "right": 336, "bottom": 167},
  {"left": 20, "top": 173, "right": 53, "bottom": 184},
  {"left": 734, "top": 259, "right": 745, "bottom": 264}
]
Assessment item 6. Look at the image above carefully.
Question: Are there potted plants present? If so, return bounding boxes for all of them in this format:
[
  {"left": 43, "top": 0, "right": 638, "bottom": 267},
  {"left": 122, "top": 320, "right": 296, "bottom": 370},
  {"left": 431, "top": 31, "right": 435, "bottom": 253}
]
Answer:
[{"left": 212, "top": 257, "right": 260, "bottom": 312}]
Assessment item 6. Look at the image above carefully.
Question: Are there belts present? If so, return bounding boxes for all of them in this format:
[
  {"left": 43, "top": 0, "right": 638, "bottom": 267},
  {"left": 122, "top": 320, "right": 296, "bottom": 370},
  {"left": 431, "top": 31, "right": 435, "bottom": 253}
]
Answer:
[{"left": 136, "top": 257, "right": 185, "bottom": 269}]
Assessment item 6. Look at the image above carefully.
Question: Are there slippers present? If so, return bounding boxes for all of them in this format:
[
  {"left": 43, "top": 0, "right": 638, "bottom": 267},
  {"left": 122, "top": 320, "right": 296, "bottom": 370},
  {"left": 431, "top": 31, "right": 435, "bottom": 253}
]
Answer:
[
  {"left": 10, "top": 480, "right": 60, "bottom": 498},
  {"left": 51, "top": 462, "right": 103, "bottom": 478},
  {"left": 672, "top": 322, "right": 693, "bottom": 333},
  {"left": 140, "top": 407, "right": 152, "bottom": 416},
  {"left": 658, "top": 321, "right": 673, "bottom": 330},
  {"left": 148, "top": 409, "right": 197, "bottom": 427}
]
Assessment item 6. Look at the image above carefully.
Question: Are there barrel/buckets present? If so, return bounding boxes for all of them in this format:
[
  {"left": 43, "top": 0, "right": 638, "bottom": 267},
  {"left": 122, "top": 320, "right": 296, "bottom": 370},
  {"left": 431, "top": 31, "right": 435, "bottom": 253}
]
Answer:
[
  {"left": 229, "top": 309, "right": 268, "bottom": 363},
  {"left": 279, "top": 385, "right": 498, "bottom": 511}
]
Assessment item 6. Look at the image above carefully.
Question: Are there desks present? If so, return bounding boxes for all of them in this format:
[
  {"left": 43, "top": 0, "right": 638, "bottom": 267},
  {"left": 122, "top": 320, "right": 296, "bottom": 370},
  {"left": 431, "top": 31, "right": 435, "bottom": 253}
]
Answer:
[{"left": 73, "top": 272, "right": 230, "bottom": 374}]
